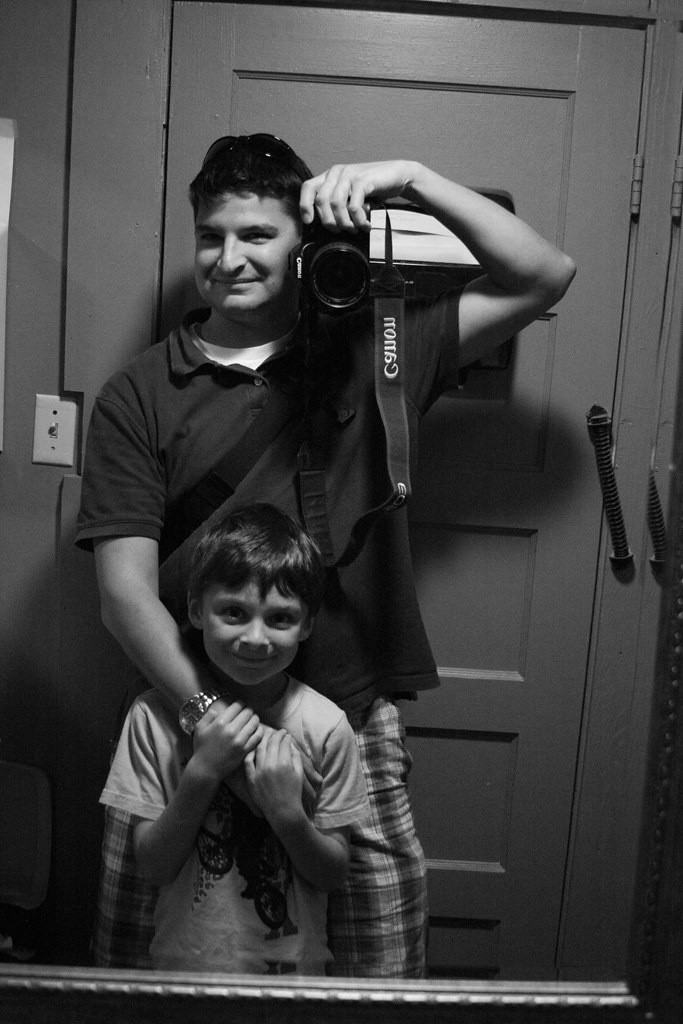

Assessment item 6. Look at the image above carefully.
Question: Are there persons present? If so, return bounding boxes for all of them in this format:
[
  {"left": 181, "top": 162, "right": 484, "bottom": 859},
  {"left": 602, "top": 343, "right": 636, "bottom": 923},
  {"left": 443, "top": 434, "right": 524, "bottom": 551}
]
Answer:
[
  {"left": 98, "top": 502, "right": 374, "bottom": 977},
  {"left": 76, "top": 132, "right": 576, "bottom": 981}
]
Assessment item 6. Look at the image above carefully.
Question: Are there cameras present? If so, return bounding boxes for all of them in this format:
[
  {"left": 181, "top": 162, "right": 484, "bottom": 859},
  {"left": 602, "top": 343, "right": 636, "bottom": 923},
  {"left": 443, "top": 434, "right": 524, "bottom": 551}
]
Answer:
[{"left": 286, "top": 198, "right": 373, "bottom": 314}]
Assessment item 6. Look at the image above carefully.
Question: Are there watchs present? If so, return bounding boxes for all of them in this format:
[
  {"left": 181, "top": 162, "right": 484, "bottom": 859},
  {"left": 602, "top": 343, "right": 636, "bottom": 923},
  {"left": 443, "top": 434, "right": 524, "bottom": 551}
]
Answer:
[{"left": 177, "top": 682, "right": 230, "bottom": 735}]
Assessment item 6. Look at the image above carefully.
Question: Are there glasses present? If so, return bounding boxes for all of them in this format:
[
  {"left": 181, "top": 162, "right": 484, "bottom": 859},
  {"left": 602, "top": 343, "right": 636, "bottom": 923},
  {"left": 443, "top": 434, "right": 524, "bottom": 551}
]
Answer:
[{"left": 202, "top": 133, "right": 308, "bottom": 183}]
